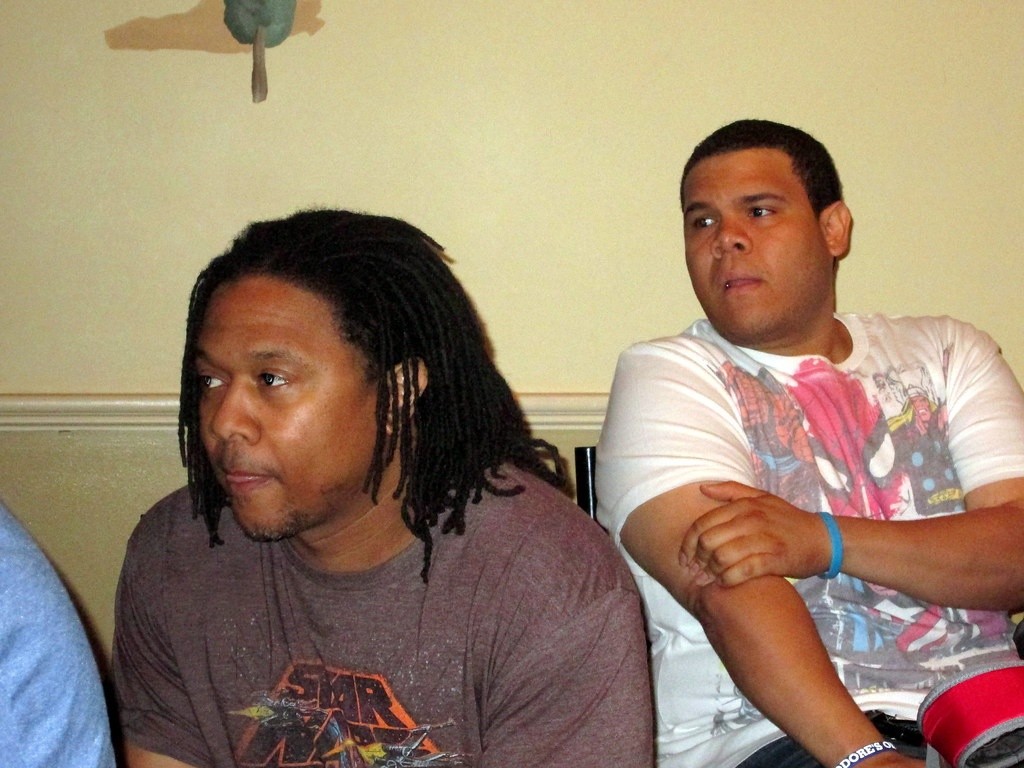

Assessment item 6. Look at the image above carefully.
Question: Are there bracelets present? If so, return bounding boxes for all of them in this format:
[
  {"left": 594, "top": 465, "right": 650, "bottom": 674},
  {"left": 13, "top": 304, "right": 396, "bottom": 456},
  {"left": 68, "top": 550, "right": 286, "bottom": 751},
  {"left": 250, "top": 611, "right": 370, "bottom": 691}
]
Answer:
[
  {"left": 814, "top": 510, "right": 844, "bottom": 579},
  {"left": 836, "top": 741, "right": 896, "bottom": 768}
]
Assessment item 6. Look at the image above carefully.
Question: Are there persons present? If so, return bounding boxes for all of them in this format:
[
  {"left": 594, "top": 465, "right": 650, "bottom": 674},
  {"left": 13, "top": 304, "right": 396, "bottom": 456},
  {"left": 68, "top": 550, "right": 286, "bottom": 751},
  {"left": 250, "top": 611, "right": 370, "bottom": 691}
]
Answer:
[
  {"left": 110, "top": 206, "right": 655, "bottom": 768},
  {"left": 0, "top": 503, "right": 115, "bottom": 768},
  {"left": 595, "top": 120, "right": 1024, "bottom": 768}
]
